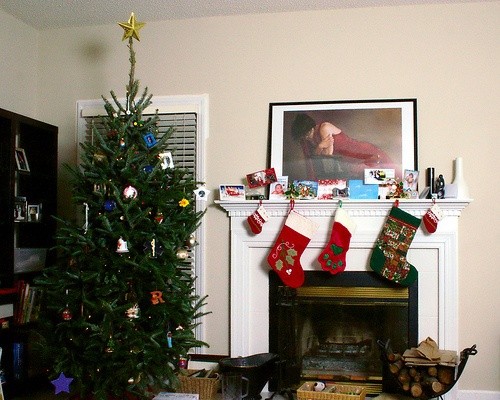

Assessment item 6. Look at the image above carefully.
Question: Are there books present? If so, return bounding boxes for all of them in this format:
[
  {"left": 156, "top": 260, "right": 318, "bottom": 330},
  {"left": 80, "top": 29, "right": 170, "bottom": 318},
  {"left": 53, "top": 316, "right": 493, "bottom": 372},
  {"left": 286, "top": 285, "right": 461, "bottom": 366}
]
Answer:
[
  {"left": 13, "top": 343, "right": 24, "bottom": 378},
  {"left": 13, "top": 278, "right": 43, "bottom": 324}
]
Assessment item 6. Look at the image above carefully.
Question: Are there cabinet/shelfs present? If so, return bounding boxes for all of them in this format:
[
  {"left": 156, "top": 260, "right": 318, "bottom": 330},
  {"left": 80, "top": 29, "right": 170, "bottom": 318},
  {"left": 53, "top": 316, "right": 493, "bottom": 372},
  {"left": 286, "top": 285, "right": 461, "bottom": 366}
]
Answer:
[{"left": 0, "top": 108, "right": 60, "bottom": 400}]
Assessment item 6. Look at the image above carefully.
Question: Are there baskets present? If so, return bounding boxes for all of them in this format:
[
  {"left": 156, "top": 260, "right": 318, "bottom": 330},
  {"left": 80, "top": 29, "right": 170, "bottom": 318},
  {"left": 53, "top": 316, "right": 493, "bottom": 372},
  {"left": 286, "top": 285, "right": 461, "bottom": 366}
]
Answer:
[
  {"left": 173, "top": 370, "right": 220, "bottom": 400},
  {"left": 297, "top": 382, "right": 367, "bottom": 400}
]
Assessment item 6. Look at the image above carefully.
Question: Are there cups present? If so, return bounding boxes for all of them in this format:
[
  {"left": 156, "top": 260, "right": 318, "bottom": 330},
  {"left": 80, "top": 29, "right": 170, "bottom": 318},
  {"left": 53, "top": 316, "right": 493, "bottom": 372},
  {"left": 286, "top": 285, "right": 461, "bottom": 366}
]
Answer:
[{"left": 221, "top": 372, "right": 249, "bottom": 400}]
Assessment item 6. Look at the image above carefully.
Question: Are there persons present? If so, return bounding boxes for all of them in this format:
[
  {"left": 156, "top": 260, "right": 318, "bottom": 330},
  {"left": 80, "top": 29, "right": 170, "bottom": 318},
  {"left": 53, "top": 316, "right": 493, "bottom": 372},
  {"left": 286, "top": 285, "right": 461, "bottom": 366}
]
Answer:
[
  {"left": 272, "top": 184, "right": 284, "bottom": 194},
  {"left": 291, "top": 112, "right": 402, "bottom": 181},
  {"left": 406, "top": 173, "right": 414, "bottom": 187}
]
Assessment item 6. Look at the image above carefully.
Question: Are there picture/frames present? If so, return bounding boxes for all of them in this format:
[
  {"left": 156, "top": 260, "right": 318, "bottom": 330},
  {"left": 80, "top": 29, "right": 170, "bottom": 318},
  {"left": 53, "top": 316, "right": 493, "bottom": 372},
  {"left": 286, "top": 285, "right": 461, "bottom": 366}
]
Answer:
[
  {"left": 15, "top": 146, "right": 30, "bottom": 172},
  {"left": 219, "top": 184, "right": 246, "bottom": 201},
  {"left": 269, "top": 176, "right": 288, "bottom": 200},
  {"left": 265, "top": 98, "right": 419, "bottom": 201},
  {"left": 13, "top": 199, "right": 39, "bottom": 222}
]
